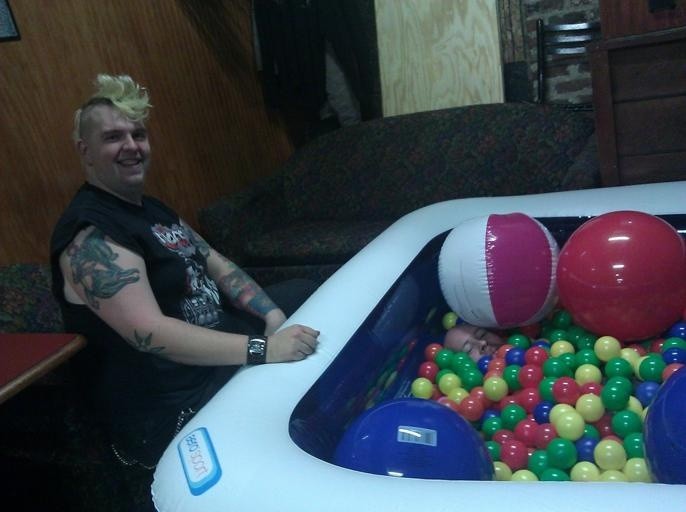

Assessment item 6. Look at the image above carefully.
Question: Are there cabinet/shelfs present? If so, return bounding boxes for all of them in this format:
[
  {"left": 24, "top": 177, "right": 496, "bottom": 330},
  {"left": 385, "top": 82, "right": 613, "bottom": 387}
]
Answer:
[{"left": 583, "top": 23, "right": 686, "bottom": 186}]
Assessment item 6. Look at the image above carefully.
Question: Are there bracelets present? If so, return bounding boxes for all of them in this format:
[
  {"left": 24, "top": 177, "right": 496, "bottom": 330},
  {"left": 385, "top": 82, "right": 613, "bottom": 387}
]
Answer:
[{"left": 247, "top": 335, "right": 268, "bottom": 366}]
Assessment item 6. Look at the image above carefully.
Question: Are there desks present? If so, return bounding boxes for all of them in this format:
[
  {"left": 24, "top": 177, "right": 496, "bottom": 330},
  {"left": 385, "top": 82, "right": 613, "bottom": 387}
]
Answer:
[{"left": 0, "top": 333, "right": 88, "bottom": 403}]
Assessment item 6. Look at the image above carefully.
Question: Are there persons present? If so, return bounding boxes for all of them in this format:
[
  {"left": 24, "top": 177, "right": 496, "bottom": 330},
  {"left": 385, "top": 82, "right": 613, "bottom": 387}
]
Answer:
[{"left": 50, "top": 73, "right": 320, "bottom": 512}]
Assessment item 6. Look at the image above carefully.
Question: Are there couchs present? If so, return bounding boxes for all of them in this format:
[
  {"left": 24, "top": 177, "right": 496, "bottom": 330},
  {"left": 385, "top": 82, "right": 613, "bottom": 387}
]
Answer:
[{"left": 196, "top": 98, "right": 600, "bottom": 286}]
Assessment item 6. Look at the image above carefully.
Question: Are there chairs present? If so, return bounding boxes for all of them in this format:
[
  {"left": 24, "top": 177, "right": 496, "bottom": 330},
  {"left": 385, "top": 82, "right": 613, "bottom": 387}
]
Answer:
[
  {"left": 0, "top": 263, "right": 152, "bottom": 506},
  {"left": 536, "top": 19, "right": 600, "bottom": 112}
]
